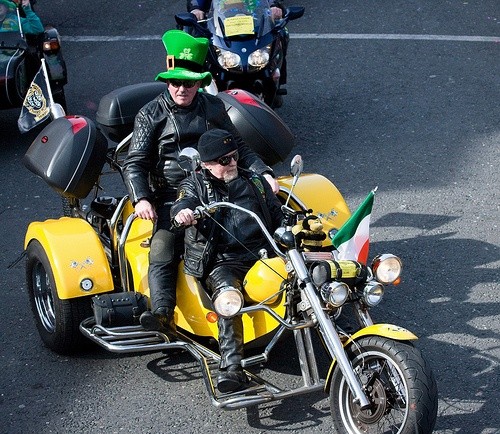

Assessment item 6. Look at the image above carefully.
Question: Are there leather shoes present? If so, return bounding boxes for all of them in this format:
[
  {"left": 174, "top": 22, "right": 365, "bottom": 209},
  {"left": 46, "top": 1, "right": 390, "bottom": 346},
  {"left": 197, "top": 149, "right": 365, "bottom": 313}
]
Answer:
[
  {"left": 139, "top": 309, "right": 177, "bottom": 341},
  {"left": 217, "top": 364, "right": 249, "bottom": 393}
]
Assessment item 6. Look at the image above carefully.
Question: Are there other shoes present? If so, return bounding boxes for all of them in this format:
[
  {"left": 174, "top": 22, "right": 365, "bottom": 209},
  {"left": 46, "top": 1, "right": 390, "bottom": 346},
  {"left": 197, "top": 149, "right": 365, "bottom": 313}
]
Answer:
[{"left": 273, "top": 67, "right": 280, "bottom": 77}]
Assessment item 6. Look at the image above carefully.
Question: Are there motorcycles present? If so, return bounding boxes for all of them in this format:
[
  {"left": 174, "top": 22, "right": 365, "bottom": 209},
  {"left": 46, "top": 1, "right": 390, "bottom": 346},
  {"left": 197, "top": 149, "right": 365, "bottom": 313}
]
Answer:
[
  {"left": 175, "top": 0, "right": 305, "bottom": 111},
  {"left": 0, "top": 9, "right": 68, "bottom": 116},
  {"left": 24, "top": 77, "right": 440, "bottom": 434}
]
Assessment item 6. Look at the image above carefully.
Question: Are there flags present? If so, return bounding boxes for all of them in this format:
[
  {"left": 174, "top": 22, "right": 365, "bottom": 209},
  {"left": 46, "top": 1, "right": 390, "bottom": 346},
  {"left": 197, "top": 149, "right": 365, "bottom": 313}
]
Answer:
[
  {"left": 331, "top": 190, "right": 374, "bottom": 267},
  {"left": 18, "top": 66, "right": 52, "bottom": 134}
]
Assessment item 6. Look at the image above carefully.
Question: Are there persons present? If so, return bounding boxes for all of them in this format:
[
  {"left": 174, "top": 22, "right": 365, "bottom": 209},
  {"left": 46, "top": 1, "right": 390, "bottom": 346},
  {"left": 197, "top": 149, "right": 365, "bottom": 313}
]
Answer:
[
  {"left": 170, "top": 129, "right": 293, "bottom": 393},
  {"left": 121, "top": 29, "right": 279, "bottom": 334},
  {"left": 186, "top": 0, "right": 286, "bottom": 24},
  {"left": 0, "top": 0, "right": 44, "bottom": 35}
]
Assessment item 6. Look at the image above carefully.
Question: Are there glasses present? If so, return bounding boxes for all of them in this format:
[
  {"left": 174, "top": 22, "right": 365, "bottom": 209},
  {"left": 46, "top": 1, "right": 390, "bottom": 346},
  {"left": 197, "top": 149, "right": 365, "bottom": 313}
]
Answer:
[
  {"left": 168, "top": 78, "right": 198, "bottom": 88},
  {"left": 213, "top": 151, "right": 239, "bottom": 166}
]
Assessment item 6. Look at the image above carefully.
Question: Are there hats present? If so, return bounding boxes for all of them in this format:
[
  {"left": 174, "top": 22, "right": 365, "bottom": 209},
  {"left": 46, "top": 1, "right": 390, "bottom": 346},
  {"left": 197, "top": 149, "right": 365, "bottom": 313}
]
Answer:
[
  {"left": 0, "top": 0, "right": 16, "bottom": 11},
  {"left": 155, "top": 30, "right": 212, "bottom": 87},
  {"left": 197, "top": 129, "right": 237, "bottom": 161}
]
